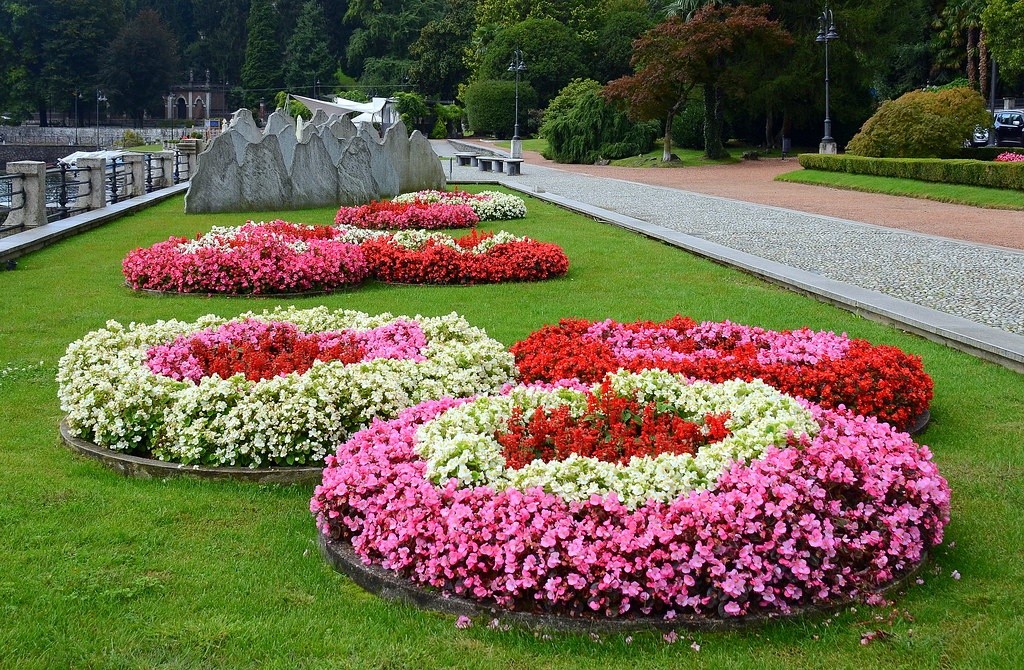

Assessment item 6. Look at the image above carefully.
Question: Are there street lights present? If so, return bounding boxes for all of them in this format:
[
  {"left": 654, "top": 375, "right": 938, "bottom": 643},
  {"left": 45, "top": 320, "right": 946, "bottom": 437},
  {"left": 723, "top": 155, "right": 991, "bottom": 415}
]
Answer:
[
  {"left": 169, "top": 94, "right": 177, "bottom": 140},
  {"left": 507, "top": 47, "right": 524, "bottom": 159},
  {"left": 73, "top": 87, "right": 83, "bottom": 143},
  {"left": 96, "top": 89, "right": 107, "bottom": 151},
  {"left": 816, "top": 1, "right": 840, "bottom": 155},
  {"left": 220, "top": 75, "right": 229, "bottom": 118},
  {"left": 313, "top": 76, "right": 320, "bottom": 98}
]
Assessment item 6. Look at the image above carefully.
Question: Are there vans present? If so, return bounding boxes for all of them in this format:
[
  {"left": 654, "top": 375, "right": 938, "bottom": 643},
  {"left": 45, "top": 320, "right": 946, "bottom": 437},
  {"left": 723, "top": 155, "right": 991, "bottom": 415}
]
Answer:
[{"left": 964, "top": 125, "right": 988, "bottom": 148}]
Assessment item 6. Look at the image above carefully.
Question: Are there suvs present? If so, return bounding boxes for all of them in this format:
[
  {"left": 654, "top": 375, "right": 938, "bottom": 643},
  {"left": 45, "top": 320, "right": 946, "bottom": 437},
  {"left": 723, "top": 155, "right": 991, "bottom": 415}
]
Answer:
[{"left": 991, "top": 108, "right": 1024, "bottom": 146}]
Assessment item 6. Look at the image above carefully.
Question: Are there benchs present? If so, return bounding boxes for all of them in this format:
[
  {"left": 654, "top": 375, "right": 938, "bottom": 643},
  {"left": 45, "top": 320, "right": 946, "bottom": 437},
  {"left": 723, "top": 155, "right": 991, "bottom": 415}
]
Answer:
[
  {"left": 453, "top": 151, "right": 481, "bottom": 167},
  {"left": 475, "top": 156, "right": 524, "bottom": 176}
]
